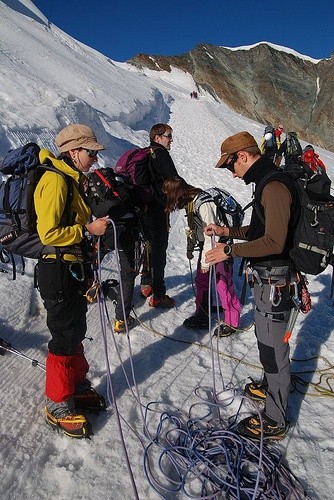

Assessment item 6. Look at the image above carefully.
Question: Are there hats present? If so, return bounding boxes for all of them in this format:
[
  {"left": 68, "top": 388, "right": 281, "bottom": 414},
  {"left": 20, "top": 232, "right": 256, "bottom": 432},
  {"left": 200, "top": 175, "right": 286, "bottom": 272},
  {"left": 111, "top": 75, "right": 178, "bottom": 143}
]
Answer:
[
  {"left": 279, "top": 125, "right": 283, "bottom": 128},
  {"left": 215, "top": 131, "right": 258, "bottom": 169},
  {"left": 54, "top": 124, "right": 106, "bottom": 153}
]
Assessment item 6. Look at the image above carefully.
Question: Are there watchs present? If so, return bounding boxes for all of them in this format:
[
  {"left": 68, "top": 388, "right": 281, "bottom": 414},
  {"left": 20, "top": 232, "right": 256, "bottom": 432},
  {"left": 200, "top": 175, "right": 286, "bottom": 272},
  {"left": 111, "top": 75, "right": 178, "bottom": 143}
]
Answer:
[{"left": 224, "top": 243, "right": 232, "bottom": 256}]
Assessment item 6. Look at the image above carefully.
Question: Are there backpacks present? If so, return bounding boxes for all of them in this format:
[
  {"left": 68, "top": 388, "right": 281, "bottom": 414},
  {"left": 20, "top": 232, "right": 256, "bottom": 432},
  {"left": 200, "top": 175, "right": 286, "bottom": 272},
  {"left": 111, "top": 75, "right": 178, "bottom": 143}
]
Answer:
[
  {"left": 0, "top": 142, "right": 75, "bottom": 260},
  {"left": 254, "top": 156, "right": 334, "bottom": 276},
  {"left": 263, "top": 125, "right": 278, "bottom": 151},
  {"left": 85, "top": 166, "right": 138, "bottom": 218},
  {"left": 286, "top": 132, "right": 303, "bottom": 155},
  {"left": 114, "top": 146, "right": 165, "bottom": 187},
  {"left": 193, "top": 187, "right": 245, "bottom": 228}
]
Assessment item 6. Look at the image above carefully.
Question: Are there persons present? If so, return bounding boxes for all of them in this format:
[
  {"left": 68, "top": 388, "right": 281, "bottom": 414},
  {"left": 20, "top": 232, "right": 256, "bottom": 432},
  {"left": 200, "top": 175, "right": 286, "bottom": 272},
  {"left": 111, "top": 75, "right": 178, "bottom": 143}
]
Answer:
[
  {"left": 204, "top": 131, "right": 302, "bottom": 441},
  {"left": 132, "top": 123, "right": 179, "bottom": 308},
  {"left": 163, "top": 174, "right": 240, "bottom": 337},
  {"left": 34, "top": 124, "right": 110, "bottom": 439},
  {"left": 191, "top": 91, "right": 197, "bottom": 98},
  {"left": 300, "top": 145, "right": 326, "bottom": 173},
  {"left": 278, "top": 131, "right": 302, "bottom": 164},
  {"left": 85, "top": 173, "right": 148, "bottom": 333},
  {"left": 260, "top": 126, "right": 283, "bottom": 160}
]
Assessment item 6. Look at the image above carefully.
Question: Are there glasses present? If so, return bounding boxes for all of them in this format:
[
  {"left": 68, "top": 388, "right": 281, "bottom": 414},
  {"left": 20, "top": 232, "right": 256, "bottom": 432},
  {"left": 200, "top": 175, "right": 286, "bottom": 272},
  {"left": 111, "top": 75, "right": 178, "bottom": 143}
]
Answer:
[
  {"left": 83, "top": 147, "right": 99, "bottom": 158},
  {"left": 162, "top": 133, "right": 172, "bottom": 139},
  {"left": 225, "top": 154, "right": 239, "bottom": 173}
]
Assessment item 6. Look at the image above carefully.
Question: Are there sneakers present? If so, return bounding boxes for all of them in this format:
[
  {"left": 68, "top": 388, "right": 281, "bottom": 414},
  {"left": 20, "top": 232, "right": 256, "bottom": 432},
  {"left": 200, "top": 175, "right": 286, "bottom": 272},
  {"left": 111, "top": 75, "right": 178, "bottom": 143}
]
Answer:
[
  {"left": 42, "top": 397, "right": 94, "bottom": 440},
  {"left": 148, "top": 293, "right": 176, "bottom": 308},
  {"left": 140, "top": 279, "right": 166, "bottom": 299},
  {"left": 244, "top": 375, "right": 269, "bottom": 403},
  {"left": 214, "top": 323, "right": 236, "bottom": 336},
  {"left": 183, "top": 314, "right": 211, "bottom": 329},
  {"left": 113, "top": 314, "right": 136, "bottom": 333},
  {"left": 237, "top": 412, "right": 289, "bottom": 442},
  {"left": 74, "top": 378, "right": 108, "bottom": 414}
]
